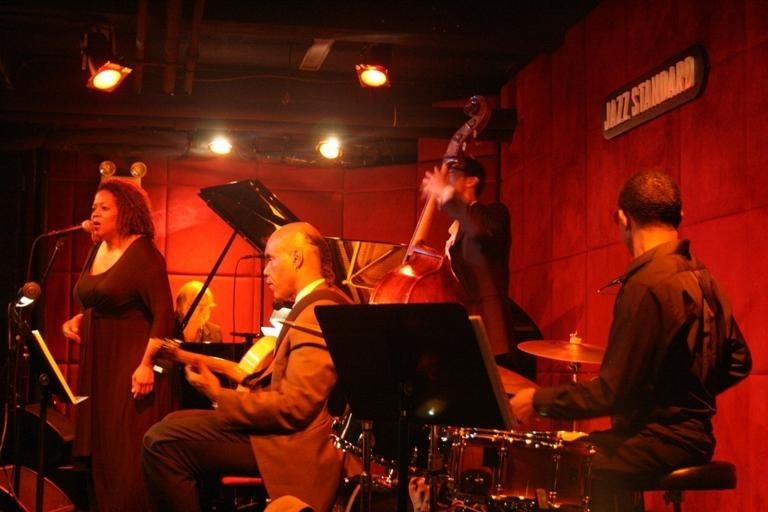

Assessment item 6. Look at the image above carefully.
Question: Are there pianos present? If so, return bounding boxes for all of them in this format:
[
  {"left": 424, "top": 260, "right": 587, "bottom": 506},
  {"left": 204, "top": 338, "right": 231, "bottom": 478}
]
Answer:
[{"left": 174, "top": 179, "right": 409, "bottom": 410}]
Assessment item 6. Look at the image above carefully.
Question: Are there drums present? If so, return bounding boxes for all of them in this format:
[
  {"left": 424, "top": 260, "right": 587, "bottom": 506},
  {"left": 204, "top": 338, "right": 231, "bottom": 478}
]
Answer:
[
  {"left": 421, "top": 426, "right": 601, "bottom": 511},
  {"left": 329, "top": 404, "right": 432, "bottom": 477}
]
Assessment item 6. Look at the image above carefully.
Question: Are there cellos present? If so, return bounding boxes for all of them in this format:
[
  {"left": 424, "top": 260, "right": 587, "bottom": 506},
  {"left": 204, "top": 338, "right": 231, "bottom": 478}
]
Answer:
[{"left": 370, "top": 96, "right": 493, "bottom": 305}]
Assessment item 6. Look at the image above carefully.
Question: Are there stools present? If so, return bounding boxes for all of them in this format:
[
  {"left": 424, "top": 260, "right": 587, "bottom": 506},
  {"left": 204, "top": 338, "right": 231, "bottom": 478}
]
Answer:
[{"left": 635, "top": 463, "right": 737, "bottom": 512}]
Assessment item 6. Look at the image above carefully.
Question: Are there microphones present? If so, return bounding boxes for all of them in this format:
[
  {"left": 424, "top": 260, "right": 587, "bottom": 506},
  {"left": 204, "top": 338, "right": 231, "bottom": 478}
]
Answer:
[{"left": 44, "top": 219, "right": 95, "bottom": 239}]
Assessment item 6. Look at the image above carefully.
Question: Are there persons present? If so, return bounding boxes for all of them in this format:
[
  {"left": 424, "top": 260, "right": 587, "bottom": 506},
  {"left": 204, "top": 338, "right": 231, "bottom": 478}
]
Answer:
[
  {"left": 141, "top": 218, "right": 357, "bottom": 512},
  {"left": 506, "top": 169, "right": 753, "bottom": 511},
  {"left": 169, "top": 280, "right": 222, "bottom": 341},
  {"left": 419, "top": 154, "right": 514, "bottom": 369},
  {"left": 63, "top": 176, "right": 178, "bottom": 512}
]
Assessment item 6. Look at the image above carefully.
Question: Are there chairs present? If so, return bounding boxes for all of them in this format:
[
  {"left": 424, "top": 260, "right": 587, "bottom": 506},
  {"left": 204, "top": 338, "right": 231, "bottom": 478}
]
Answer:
[{"left": 221, "top": 410, "right": 352, "bottom": 512}]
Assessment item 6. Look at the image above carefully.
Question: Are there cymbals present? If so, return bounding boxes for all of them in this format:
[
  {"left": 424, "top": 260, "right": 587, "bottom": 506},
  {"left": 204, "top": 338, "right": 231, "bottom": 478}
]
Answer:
[
  {"left": 495, "top": 364, "right": 539, "bottom": 395},
  {"left": 517, "top": 340, "right": 603, "bottom": 365}
]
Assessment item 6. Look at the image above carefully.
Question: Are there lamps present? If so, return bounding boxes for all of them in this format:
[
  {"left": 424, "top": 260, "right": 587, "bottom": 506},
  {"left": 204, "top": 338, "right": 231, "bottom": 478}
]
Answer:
[
  {"left": 81, "top": 38, "right": 134, "bottom": 95},
  {"left": 353, "top": 46, "right": 397, "bottom": 93}
]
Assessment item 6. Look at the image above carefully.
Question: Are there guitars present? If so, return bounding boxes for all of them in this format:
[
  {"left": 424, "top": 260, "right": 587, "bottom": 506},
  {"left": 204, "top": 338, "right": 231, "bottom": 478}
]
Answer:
[{"left": 153, "top": 336, "right": 280, "bottom": 393}]
